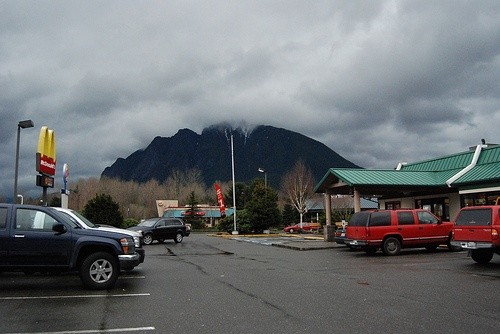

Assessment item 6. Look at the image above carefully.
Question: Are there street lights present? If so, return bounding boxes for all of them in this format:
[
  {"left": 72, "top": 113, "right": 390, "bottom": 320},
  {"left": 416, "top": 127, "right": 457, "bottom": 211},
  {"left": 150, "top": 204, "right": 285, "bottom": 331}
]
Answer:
[
  {"left": 13, "top": 120, "right": 35, "bottom": 203},
  {"left": 258, "top": 168, "right": 267, "bottom": 201},
  {"left": 224, "top": 127, "right": 238, "bottom": 234}
]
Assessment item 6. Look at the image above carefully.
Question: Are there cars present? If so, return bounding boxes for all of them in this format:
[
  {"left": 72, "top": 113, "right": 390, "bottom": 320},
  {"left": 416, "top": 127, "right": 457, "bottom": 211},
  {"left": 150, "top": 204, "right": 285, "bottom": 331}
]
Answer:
[
  {"left": 284, "top": 222, "right": 321, "bottom": 234},
  {"left": 125, "top": 217, "right": 191, "bottom": 245}
]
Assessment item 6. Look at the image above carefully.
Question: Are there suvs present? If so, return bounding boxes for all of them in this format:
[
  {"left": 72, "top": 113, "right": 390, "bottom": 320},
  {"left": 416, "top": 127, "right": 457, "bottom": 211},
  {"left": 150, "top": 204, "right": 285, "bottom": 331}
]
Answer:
[
  {"left": 0, "top": 203, "right": 141, "bottom": 290},
  {"left": 335, "top": 209, "right": 454, "bottom": 255},
  {"left": 16, "top": 207, "right": 145, "bottom": 270},
  {"left": 448, "top": 205, "right": 500, "bottom": 264}
]
